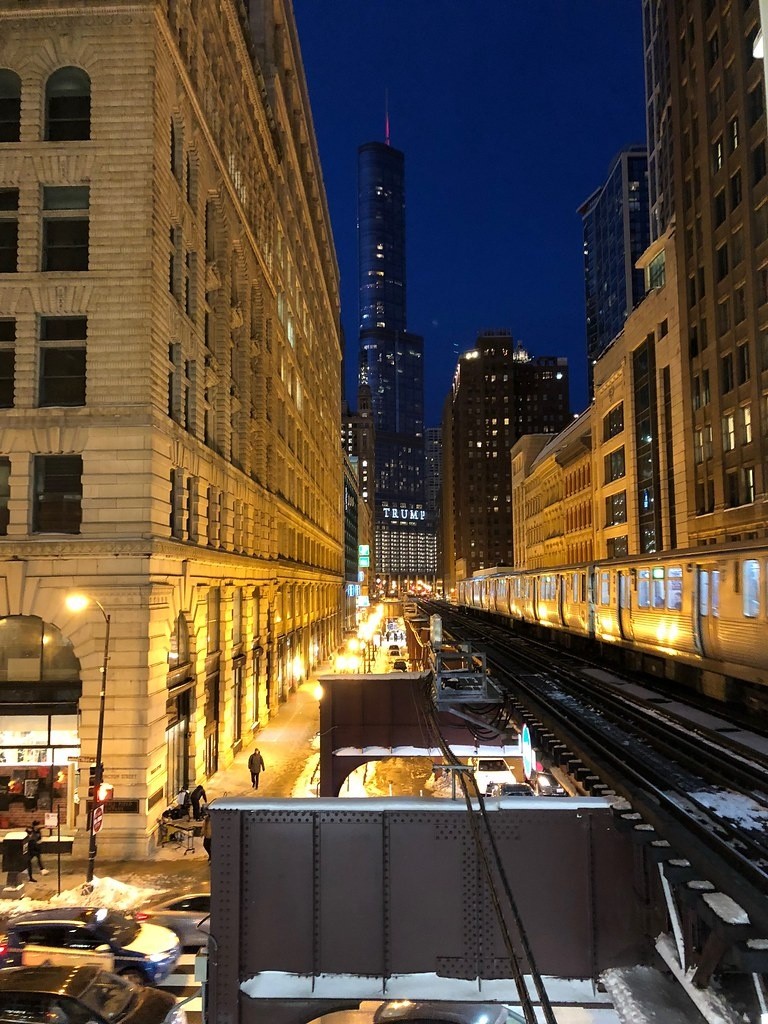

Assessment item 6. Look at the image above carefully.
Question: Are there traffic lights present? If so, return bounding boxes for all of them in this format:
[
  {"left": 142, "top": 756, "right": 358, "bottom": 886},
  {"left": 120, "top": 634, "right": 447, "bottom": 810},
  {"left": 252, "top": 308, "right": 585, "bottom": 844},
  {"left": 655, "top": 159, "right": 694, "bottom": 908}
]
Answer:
[{"left": 97, "top": 785, "right": 115, "bottom": 805}]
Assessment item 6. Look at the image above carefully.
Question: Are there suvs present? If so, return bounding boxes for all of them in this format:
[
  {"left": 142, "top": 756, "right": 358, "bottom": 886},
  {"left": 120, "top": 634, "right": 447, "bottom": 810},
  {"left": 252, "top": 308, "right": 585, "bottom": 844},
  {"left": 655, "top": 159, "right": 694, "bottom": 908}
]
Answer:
[{"left": 0, "top": 906, "right": 183, "bottom": 989}]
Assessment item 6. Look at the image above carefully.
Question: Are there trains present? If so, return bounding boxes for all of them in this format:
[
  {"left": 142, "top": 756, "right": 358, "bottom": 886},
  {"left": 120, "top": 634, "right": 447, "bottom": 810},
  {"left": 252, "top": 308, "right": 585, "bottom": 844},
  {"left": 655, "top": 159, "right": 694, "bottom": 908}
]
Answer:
[{"left": 455, "top": 537, "right": 768, "bottom": 713}]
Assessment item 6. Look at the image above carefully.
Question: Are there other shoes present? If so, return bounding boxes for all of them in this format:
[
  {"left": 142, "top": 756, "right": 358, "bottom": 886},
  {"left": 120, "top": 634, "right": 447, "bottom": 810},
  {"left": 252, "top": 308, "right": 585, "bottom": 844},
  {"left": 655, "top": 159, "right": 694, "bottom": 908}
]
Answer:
[{"left": 252, "top": 783, "right": 259, "bottom": 790}]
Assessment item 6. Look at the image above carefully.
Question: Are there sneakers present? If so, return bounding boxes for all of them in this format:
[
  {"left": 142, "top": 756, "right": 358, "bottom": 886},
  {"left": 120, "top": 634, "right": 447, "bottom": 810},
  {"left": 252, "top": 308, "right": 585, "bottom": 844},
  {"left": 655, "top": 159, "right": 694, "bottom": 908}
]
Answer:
[
  {"left": 40, "top": 868, "right": 50, "bottom": 876},
  {"left": 27, "top": 878, "right": 38, "bottom": 884}
]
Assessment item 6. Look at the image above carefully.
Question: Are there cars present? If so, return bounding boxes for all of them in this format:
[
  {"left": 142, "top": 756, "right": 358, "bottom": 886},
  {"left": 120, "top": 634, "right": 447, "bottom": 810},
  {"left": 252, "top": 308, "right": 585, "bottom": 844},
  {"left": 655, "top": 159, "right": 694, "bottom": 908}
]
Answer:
[
  {"left": 466, "top": 756, "right": 565, "bottom": 797},
  {"left": 134, "top": 892, "right": 211, "bottom": 950},
  {"left": 0, "top": 963, "right": 188, "bottom": 1024},
  {"left": 387, "top": 645, "right": 408, "bottom": 674}
]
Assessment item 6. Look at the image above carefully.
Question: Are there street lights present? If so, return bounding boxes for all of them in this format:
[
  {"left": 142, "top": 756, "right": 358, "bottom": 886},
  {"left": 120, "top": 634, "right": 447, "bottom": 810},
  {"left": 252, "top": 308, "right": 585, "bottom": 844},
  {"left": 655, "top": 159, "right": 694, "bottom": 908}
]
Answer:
[
  {"left": 67, "top": 592, "right": 112, "bottom": 885},
  {"left": 334, "top": 601, "right": 389, "bottom": 672}
]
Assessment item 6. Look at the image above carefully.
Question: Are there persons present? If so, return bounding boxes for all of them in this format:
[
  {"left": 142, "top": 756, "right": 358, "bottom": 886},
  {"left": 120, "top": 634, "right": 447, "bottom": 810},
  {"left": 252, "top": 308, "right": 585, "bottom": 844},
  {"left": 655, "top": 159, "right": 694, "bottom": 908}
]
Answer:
[
  {"left": 30, "top": 820, "right": 49, "bottom": 875},
  {"left": 248, "top": 749, "right": 265, "bottom": 790},
  {"left": 191, "top": 785, "right": 207, "bottom": 821},
  {"left": 25, "top": 828, "right": 37, "bottom": 882},
  {"left": 180, "top": 786, "right": 190, "bottom": 811},
  {"left": 200, "top": 814, "right": 211, "bottom": 865}
]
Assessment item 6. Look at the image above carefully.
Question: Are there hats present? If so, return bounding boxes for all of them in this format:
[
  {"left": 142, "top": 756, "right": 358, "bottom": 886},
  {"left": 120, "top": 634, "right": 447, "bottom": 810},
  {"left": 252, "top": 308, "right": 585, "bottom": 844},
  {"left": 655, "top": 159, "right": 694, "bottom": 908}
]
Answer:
[
  {"left": 255, "top": 748, "right": 259, "bottom": 751},
  {"left": 25, "top": 828, "right": 35, "bottom": 834},
  {"left": 32, "top": 820, "right": 41, "bottom": 827}
]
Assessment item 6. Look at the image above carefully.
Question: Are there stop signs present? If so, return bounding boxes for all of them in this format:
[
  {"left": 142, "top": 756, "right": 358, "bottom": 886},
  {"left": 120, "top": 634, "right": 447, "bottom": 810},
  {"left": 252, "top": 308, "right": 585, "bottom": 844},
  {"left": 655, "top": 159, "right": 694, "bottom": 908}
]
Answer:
[{"left": 92, "top": 803, "right": 104, "bottom": 836}]
